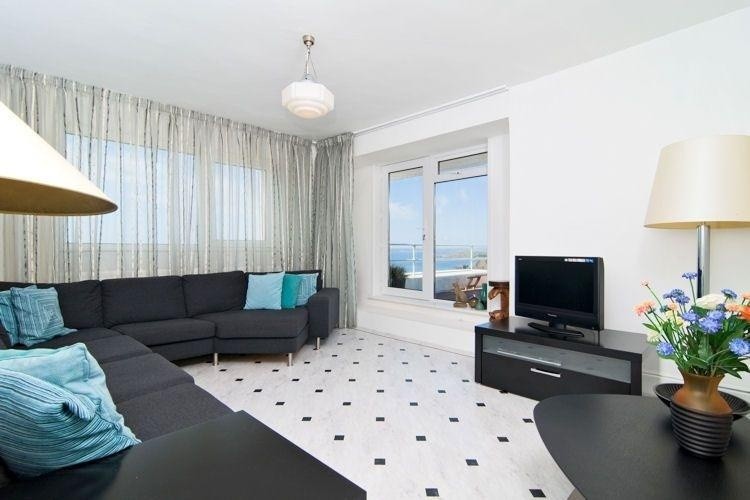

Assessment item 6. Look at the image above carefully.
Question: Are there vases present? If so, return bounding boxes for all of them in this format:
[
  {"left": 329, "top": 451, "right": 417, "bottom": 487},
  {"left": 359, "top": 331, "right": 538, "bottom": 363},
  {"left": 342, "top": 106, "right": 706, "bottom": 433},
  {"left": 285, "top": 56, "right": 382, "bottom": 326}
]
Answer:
[{"left": 664, "top": 364, "right": 735, "bottom": 460}]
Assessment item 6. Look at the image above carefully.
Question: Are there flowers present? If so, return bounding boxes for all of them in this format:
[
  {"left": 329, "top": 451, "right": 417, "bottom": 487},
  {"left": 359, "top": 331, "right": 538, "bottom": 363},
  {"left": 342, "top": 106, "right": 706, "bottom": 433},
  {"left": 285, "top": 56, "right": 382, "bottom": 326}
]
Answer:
[{"left": 632, "top": 269, "right": 749, "bottom": 382}]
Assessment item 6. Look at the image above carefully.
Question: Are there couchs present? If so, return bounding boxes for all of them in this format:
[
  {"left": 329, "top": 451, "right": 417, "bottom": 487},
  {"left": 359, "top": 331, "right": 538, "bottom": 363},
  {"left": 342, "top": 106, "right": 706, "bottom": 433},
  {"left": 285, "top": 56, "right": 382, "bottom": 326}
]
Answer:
[{"left": 0, "top": 269, "right": 370, "bottom": 500}]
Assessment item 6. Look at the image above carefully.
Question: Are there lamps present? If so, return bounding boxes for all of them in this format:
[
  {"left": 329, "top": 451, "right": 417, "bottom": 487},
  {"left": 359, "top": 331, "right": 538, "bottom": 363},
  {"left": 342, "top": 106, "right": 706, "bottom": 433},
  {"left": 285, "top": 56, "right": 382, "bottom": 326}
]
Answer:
[
  {"left": 640, "top": 133, "right": 749, "bottom": 300},
  {"left": 278, "top": 33, "right": 335, "bottom": 122}
]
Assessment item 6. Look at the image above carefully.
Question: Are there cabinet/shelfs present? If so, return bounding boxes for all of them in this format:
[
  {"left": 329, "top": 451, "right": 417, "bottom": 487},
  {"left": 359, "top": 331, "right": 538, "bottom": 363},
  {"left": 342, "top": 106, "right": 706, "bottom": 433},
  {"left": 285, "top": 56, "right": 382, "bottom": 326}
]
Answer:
[{"left": 472, "top": 315, "right": 647, "bottom": 412}]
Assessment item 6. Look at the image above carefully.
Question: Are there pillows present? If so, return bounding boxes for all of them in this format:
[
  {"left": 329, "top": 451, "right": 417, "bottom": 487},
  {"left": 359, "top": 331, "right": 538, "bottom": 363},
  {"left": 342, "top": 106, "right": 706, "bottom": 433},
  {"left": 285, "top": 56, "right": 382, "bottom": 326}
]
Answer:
[
  {"left": 0, "top": 343, "right": 143, "bottom": 477},
  {"left": 0, "top": 285, "right": 77, "bottom": 349},
  {"left": 241, "top": 268, "right": 322, "bottom": 315}
]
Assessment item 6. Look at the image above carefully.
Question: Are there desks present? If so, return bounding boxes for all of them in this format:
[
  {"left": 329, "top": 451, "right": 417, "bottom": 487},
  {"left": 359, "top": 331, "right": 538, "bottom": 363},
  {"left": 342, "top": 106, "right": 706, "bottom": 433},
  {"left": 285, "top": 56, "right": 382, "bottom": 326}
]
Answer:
[{"left": 532, "top": 388, "right": 750, "bottom": 499}]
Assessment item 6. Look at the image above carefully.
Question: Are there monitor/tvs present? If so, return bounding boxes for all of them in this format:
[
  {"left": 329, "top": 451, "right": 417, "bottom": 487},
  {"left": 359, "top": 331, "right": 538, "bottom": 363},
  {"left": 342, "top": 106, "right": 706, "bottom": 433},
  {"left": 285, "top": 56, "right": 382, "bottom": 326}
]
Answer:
[{"left": 514, "top": 256, "right": 604, "bottom": 338}]
